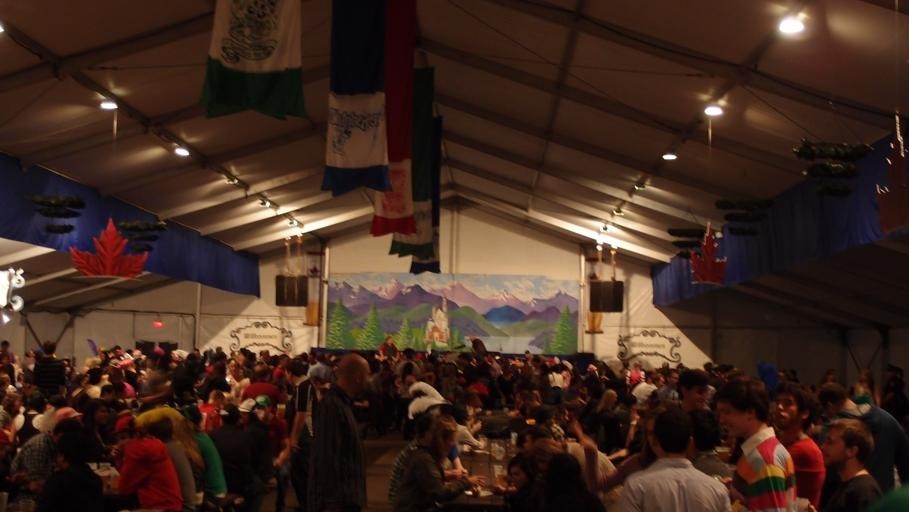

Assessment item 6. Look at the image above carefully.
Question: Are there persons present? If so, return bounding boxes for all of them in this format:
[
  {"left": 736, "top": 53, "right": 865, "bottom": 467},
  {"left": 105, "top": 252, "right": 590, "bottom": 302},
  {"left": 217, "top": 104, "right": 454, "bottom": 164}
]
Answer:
[{"left": 1, "top": 337, "right": 909, "bottom": 512}]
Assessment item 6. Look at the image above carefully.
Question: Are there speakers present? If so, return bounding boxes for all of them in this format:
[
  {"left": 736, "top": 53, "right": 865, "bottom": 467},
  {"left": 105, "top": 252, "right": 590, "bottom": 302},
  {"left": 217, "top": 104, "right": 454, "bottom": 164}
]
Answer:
[
  {"left": 275, "top": 275, "right": 309, "bottom": 307},
  {"left": 590, "top": 280, "right": 624, "bottom": 313}
]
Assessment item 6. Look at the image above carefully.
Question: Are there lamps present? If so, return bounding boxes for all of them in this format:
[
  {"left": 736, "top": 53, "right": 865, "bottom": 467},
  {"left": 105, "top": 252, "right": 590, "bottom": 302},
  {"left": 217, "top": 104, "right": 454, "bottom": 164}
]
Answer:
[
  {"left": 602, "top": 3, "right": 817, "bottom": 232},
  {"left": 87, "top": 82, "right": 303, "bottom": 228}
]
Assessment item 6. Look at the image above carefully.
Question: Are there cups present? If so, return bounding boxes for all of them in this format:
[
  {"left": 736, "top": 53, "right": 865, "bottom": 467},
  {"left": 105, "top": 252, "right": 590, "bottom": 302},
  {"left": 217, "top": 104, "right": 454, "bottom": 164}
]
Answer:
[{"left": 18, "top": 499, "right": 35, "bottom": 512}]
